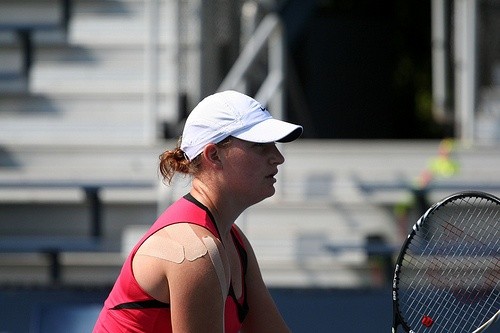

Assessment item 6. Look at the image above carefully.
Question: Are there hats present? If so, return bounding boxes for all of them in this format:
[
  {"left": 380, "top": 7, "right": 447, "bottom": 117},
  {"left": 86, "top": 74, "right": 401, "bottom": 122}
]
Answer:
[{"left": 179, "top": 90, "right": 304, "bottom": 163}]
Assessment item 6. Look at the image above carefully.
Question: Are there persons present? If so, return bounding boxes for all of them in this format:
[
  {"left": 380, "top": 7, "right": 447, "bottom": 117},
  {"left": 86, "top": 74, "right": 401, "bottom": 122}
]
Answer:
[{"left": 90, "top": 90, "right": 304, "bottom": 332}]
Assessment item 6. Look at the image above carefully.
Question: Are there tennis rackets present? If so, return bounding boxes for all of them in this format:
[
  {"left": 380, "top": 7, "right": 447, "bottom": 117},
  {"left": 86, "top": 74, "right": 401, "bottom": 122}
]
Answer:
[{"left": 392, "top": 190, "right": 500, "bottom": 333}]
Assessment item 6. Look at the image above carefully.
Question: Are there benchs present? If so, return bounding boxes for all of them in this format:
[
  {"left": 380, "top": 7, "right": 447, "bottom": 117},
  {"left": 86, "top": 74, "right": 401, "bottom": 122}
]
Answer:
[{"left": 0, "top": 174, "right": 160, "bottom": 289}]
